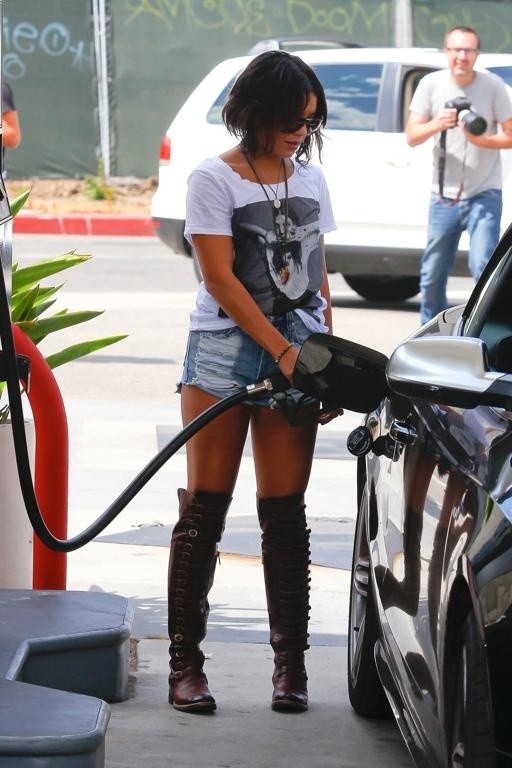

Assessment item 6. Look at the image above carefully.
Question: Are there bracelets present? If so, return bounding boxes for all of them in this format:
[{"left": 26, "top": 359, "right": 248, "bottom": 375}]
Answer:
[{"left": 276, "top": 343, "right": 295, "bottom": 365}]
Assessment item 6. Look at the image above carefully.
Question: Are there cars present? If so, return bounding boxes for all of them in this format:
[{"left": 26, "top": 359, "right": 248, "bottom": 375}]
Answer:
[{"left": 347, "top": 224, "right": 512, "bottom": 767}]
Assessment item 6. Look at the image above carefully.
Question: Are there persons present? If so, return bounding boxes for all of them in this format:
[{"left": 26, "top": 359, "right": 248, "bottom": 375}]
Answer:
[
  {"left": 237, "top": 204, "right": 322, "bottom": 316},
  {"left": 1, "top": 80, "right": 23, "bottom": 176},
  {"left": 404, "top": 25, "right": 512, "bottom": 330},
  {"left": 164, "top": 48, "right": 346, "bottom": 713}
]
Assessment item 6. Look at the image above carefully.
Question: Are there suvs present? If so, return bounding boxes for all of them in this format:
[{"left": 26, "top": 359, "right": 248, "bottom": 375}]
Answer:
[{"left": 150, "top": 37, "right": 512, "bottom": 301}]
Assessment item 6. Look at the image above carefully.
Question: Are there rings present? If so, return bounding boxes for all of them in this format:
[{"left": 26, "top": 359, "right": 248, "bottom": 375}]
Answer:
[{"left": 334, "top": 406, "right": 344, "bottom": 417}]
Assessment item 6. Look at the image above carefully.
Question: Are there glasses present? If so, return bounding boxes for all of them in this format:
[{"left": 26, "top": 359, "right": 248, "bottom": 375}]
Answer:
[
  {"left": 445, "top": 45, "right": 479, "bottom": 56},
  {"left": 275, "top": 112, "right": 323, "bottom": 137}
]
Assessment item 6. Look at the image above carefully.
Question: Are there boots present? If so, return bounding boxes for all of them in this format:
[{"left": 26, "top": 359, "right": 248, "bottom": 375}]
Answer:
[
  {"left": 256, "top": 490, "right": 310, "bottom": 712},
  {"left": 168, "top": 488, "right": 234, "bottom": 711}
]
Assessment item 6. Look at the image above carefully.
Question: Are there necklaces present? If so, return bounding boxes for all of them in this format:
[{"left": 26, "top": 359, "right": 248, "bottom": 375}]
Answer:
[
  {"left": 241, "top": 142, "right": 289, "bottom": 285},
  {"left": 247, "top": 152, "right": 284, "bottom": 210}
]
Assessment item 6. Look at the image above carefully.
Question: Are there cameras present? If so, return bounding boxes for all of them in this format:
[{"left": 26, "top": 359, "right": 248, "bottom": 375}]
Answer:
[{"left": 443, "top": 95, "right": 488, "bottom": 137}]
